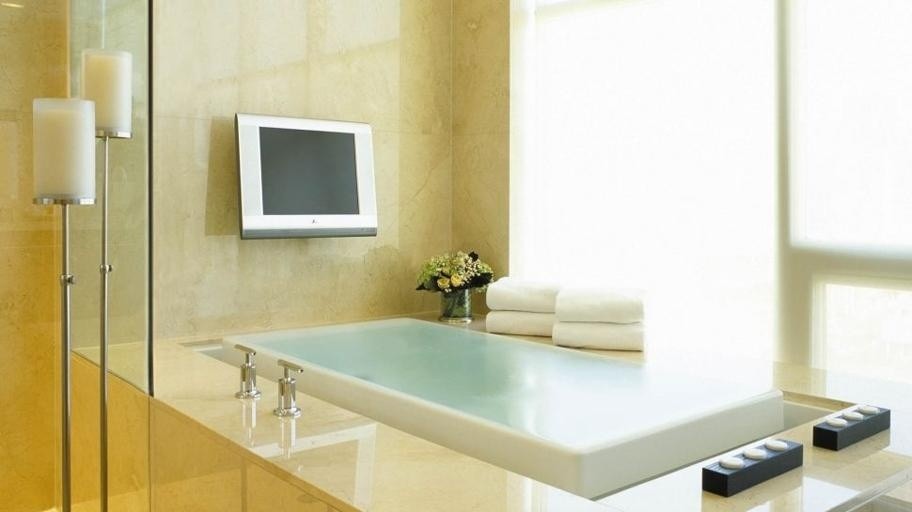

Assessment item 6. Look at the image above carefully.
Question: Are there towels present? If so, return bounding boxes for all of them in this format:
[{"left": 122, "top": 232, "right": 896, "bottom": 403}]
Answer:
[{"left": 486, "top": 276, "right": 646, "bottom": 351}]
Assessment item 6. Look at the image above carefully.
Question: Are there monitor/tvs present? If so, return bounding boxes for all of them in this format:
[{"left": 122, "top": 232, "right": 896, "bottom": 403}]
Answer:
[{"left": 234, "top": 113, "right": 377, "bottom": 239}]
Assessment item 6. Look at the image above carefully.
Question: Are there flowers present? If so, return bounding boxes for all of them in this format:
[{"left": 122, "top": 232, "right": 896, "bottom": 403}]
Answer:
[{"left": 415, "top": 249, "right": 494, "bottom": 317}]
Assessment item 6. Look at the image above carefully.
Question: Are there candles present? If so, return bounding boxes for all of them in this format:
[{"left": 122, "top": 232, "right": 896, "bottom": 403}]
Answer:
[{"left": 32, "top": 49, "right": 133, "bottom": 200}]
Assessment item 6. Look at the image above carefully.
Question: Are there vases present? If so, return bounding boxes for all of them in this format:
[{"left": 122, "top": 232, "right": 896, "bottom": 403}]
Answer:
[{"left": 439, "top": 288, "right": 475, "bottom": 325}]
